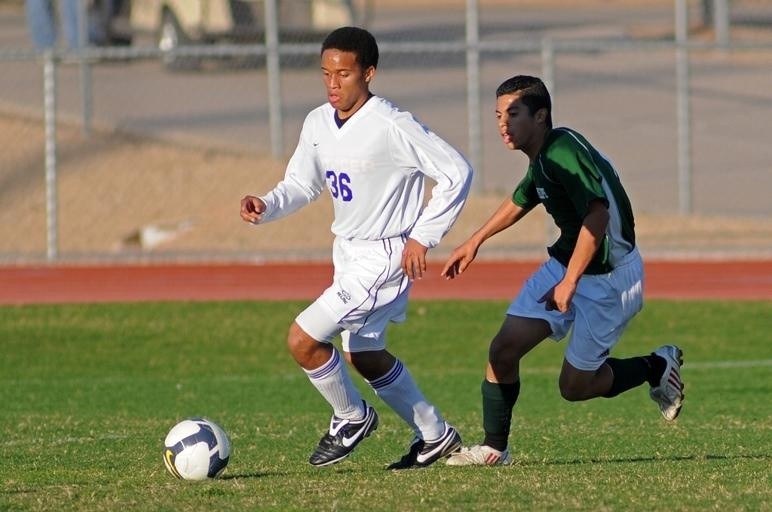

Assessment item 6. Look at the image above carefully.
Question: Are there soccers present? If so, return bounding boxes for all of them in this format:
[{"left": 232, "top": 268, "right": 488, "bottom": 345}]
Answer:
[{"left": 162, "top": 417, "right": 231, "bottom": 480}]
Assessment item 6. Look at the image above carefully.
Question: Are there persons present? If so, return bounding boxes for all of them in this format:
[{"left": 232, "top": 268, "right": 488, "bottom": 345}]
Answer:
[
  {"left": 239, "top": 26, "right": 472, "bottom": 471},
  {"left": 439, "top": 74, "right": 685, "bottom": 468}
]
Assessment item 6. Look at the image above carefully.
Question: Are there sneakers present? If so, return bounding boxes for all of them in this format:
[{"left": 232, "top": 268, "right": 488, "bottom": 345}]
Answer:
[
  {"left": 385, "top": 425, "right": 463, "bottom": 470},
  {"left": 649, "top": 345, "right": 684, "bottom": 421},
  {"left": 445, "top": 443, "right": 513, "bottom": 467},
  {"left": 308, "top": 399, "right": 378, "bottom": 468}
]
